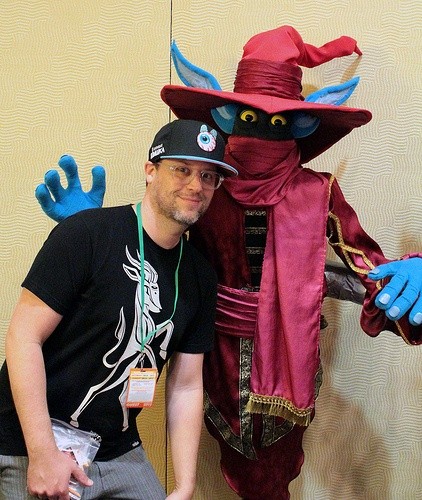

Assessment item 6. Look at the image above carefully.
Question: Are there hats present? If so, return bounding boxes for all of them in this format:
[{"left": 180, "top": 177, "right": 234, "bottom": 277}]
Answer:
[{"left": 149, "top": 120, "right": 238, "bottom": 178}]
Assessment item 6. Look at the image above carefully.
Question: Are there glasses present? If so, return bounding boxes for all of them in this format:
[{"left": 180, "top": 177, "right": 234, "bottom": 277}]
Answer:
[{"left": 157, "top": 162, "right": 224, "bottom": 189}]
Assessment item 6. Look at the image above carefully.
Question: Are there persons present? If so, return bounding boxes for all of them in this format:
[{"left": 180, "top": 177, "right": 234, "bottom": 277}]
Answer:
[
  {"left": 34, "top": 25, "right": 422, "bottom": 500},
  {"left": 0, "top": 120, "right": 237, "bottom": 500}
]
